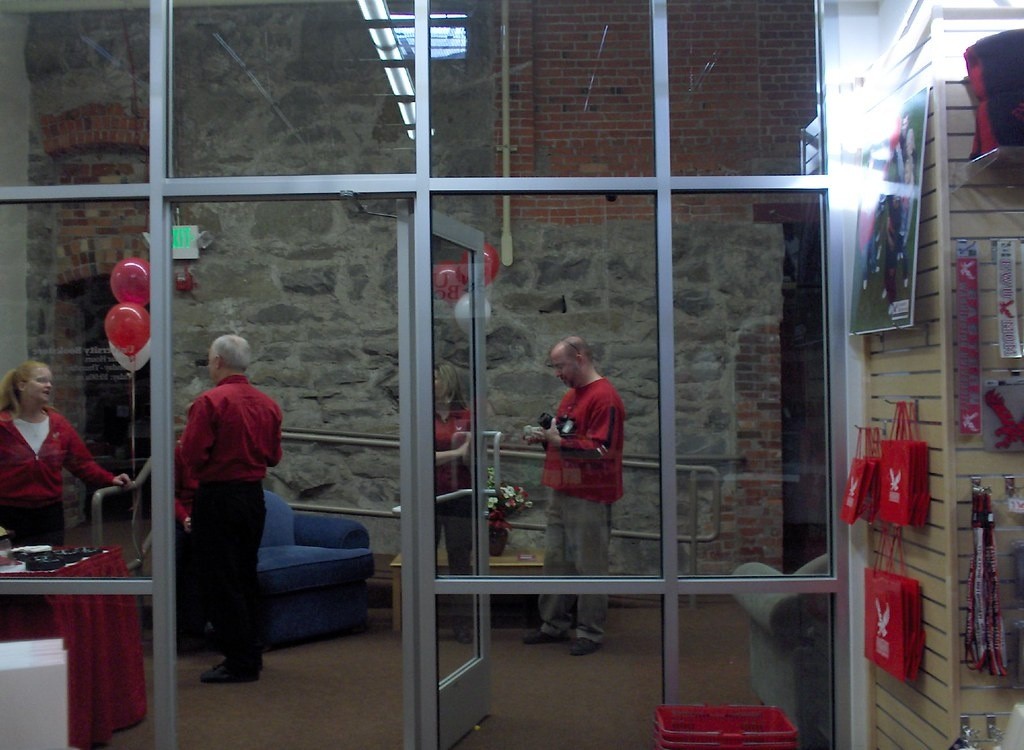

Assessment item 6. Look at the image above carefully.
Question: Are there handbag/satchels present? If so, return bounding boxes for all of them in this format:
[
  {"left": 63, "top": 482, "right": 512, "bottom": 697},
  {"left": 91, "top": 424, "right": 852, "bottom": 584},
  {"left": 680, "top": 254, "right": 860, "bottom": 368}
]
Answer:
[
  {"left": 841, "top": 426, "right": 884, "bottom": 523},
  {"left": 877, "top": 401, "right": 930, "bottom": 528},
  {"left": 863, "top": 523, "right": 923, "bottom": 682}
]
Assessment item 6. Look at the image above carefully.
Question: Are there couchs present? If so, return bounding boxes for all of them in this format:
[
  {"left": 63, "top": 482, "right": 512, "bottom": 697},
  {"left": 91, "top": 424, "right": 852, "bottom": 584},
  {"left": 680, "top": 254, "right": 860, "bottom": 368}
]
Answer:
[
  {"left": 731, "top": 554, "right": 830, "bottom": 750},
  {"left": 257, "top": 489, "right": 376, "bottom": 654}
]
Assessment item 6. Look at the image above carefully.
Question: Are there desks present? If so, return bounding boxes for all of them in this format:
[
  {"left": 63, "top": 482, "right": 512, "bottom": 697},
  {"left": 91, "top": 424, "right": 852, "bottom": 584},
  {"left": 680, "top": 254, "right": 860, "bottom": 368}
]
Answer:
[{"left": 0, "top": 544, "right": 146, "bottom": 750}]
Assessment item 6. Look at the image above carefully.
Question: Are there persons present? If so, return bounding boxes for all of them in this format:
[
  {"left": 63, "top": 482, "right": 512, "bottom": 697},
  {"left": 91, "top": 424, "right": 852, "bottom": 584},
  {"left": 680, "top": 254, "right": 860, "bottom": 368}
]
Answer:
[
  {"left": 434, "top": 362, "right": 474, "bottom": 642},
  {"left": 858, "top": 113, "right": 918, "bottom": 304},
  {"left": 175, "top": 335, "right": 283, "bottom": 683},
  {"left": 523, "top": 337, "right": 626, "bottom": 654},
  {"left": 0, "top": 359, "right": 132, "bottom": 547}
]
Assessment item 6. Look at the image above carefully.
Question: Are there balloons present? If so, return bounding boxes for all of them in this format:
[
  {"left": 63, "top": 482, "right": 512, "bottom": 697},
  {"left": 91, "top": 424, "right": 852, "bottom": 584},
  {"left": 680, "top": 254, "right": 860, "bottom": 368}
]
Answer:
[
  {"left": 110, "top": 257, "right": 151, "bottom": 306},
  {"left": 455, "top": 293, "right": 491, "bottom": 333},
  {"left": 432, "top": 260, "right": 465, "bottom": 306},
  {"left": 105, "top": 302, "right": 151, "bottom": 358},
  {"left": 460, "top": 242, "right": 499, "bottom": 286}
]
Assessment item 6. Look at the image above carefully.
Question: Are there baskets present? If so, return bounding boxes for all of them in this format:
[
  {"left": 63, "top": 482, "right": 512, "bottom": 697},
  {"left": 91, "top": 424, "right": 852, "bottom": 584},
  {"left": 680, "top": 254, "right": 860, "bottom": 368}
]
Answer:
[{"left": 653, "top": 704, "right": 799, "bottom": 750}]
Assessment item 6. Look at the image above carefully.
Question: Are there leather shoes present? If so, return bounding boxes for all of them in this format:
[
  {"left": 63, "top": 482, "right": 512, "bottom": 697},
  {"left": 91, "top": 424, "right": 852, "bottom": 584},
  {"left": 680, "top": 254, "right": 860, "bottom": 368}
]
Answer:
[
  {"left": 214, "top": 662, "right": 264, "bottom": 671},
  {"left": 201, "top": 664, "right": 258, "bottom": 682}
]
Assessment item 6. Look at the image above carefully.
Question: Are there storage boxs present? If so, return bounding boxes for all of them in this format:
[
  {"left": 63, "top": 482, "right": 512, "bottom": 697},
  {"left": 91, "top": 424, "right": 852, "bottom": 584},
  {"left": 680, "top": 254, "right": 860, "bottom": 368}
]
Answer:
[{"left": 0, "top": 638, "right": 69, "bottom": 750}]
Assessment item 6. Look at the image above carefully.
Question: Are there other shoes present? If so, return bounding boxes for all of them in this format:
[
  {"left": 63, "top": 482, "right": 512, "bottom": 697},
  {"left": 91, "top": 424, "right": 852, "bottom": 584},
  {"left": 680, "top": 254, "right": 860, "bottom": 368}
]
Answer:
[
  {"left": 522, "top": 630, "right": 572, "bottom": 643},
  {"left": 571, "top": 638, "right": 600, "bottom": 655}
]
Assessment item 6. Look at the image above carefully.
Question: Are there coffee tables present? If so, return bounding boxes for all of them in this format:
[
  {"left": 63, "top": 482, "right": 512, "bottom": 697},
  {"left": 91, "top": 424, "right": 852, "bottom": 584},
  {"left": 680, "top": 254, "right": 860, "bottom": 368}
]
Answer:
[{"left": 390, "top": 546, "right": 545, "bottom": 634}]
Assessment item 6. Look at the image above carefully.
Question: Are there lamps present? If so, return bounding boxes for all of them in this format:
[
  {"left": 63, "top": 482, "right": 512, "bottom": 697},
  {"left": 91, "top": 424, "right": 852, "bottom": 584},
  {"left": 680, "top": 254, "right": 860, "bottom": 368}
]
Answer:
[
  {"left": 455, "top": 294, "right": 492, "bottom": 335},
  {"left": 357, "top": 0, "right": 436, "bottom": 139},
  {"left": 141, "top": 224, "right": 213, "bottom": 260}
]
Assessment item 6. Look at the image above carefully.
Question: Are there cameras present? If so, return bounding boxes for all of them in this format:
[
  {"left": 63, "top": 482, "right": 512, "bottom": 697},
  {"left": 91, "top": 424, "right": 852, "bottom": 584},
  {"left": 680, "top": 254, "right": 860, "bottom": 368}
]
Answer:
[{"left": 538, "top": 412, "right": 576, "bottom": 437}]
